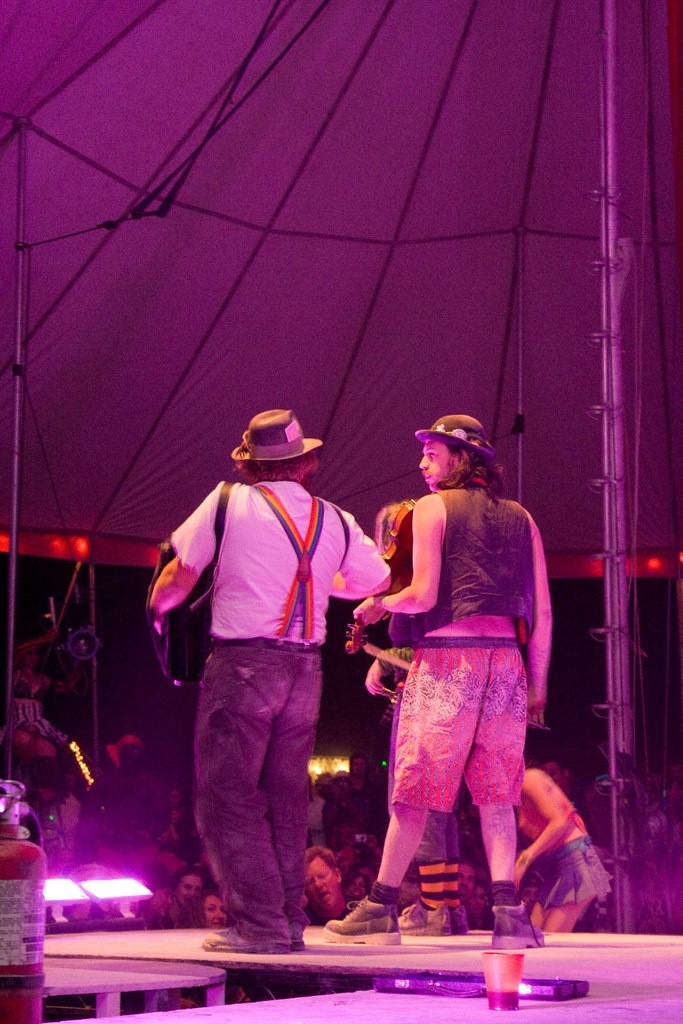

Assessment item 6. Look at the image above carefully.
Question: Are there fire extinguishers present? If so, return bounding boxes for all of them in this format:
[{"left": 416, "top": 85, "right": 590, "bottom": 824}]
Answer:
[{"left": 0, "top": 779, "right": 49, "bottom": 1024}]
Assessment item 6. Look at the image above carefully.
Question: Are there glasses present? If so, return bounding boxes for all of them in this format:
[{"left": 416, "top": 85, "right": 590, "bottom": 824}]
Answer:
[{"left": 303, "top": 868, "right": 334, "bottom": 887}]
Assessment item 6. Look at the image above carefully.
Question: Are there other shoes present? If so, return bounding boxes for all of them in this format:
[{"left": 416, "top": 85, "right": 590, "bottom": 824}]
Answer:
[
  {"left": 447, "top": 906, "right": 470, "bottom": 933},
  {"left": 492, "top": 902, "right": 547, "bottom": 951},
  {"left": 290, "top": 938, "right": 308, "bottom": 951},
  {"left": 390, "top": 906, "right": 452, "bottom": 935},
  {"left": 203, "top": 923, "right": 291, "bottom": 953},
  {"left": 322, "top": 898, "right": 404, "bottom": 946}
]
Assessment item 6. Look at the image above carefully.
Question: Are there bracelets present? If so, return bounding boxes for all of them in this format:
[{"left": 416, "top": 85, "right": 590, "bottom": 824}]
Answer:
[{"left": 373, "top": 595, "right": 389, "bottom": 619}]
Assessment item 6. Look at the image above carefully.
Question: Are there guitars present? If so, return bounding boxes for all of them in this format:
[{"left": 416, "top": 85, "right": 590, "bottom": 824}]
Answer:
[{"left": 347, "top": 624, "right": 412, "bottom": 670}]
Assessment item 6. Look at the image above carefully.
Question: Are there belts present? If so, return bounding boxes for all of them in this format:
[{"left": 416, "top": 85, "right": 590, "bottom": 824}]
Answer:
[{"left": 553, "top": 834, "right": 595, "bottom": 864}]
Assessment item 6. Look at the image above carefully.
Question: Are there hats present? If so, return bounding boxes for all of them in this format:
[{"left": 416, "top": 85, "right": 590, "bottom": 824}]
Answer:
[
  {"left": 414, "top": 415, "right": 496, "bottom": 458},
  {"left": 232, "top": 410, "right": 324, "bottom": 464}
]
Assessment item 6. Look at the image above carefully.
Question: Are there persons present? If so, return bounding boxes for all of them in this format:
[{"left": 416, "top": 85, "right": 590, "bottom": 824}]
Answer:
[
  {"left": 322, "top": 414, "right": 553, "bottom": 949},
  {"left": 5, "top": 629, "right": 616, "bottom": 934},
  {"left": 148, "top": 408, "right": 390, "bottom": 954},
  {"left": 365, "top": 499, "right": 467, "bottom": 937}
]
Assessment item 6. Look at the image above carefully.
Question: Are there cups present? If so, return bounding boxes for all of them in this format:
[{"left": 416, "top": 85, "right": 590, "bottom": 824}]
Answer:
[{"left": 485, "top": 951, "right": 524, "bottom": 1011}]
